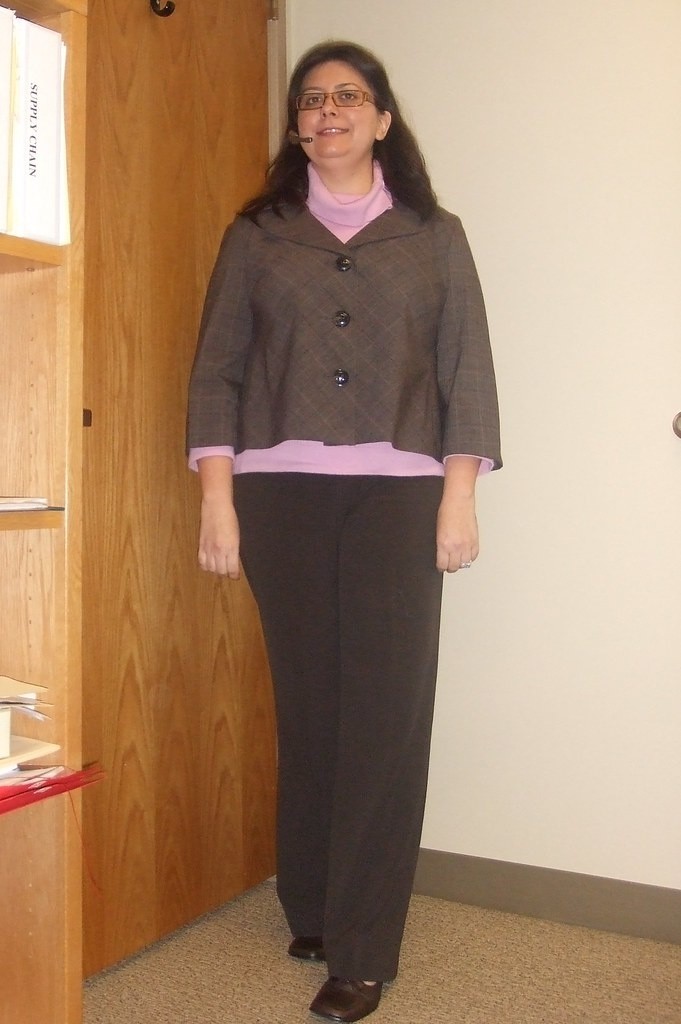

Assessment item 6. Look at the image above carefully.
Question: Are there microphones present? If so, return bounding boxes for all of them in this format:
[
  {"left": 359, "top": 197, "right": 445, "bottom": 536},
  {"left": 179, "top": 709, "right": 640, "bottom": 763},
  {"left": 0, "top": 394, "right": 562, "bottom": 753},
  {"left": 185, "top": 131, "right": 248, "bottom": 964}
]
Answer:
[{"left": 288, "top": 131, "right": 312, "bottom": 144}]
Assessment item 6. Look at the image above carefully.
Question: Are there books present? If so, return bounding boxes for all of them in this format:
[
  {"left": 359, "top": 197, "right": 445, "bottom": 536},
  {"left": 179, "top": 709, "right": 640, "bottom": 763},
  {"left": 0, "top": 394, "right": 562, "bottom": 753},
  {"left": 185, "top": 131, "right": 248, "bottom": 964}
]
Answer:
[
  {"left": 0, "top": 497, "right": 48, "bottom": 509},
  {"left": 1, "top": 676, "right": 65, "bottom": 786}
]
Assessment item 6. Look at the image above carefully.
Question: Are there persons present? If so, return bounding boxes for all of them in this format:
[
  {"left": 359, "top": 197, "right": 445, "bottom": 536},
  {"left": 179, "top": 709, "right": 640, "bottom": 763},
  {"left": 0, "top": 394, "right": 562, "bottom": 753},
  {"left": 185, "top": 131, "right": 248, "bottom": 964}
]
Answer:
[{"left": 187, "top": 41, "right": 502, "bottom": 1020}]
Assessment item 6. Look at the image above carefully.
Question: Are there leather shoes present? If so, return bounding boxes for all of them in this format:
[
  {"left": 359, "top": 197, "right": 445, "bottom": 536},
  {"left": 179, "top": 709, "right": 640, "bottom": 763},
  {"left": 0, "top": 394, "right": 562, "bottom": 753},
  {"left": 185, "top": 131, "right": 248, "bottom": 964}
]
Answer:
[
  {"left": 310, "top": 977, "right": 383, "bottom": 1022},
  {"left": 289, "top": 936, "right": 324, "bottom": 961}
]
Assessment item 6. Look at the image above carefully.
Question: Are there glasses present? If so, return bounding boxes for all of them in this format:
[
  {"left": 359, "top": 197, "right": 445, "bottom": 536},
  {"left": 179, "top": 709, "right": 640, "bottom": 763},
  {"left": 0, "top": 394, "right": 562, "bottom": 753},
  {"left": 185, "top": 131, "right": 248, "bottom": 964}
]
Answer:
[{"left": 295, "top": 91, "right": 380, "bottom": 110}]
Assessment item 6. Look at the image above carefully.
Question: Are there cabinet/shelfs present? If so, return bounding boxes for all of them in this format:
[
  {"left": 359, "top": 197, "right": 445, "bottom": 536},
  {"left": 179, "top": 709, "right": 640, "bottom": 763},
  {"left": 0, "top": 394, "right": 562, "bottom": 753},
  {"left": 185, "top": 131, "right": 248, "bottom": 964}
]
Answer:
[{"left": 2, "top": 2, "right": 107, "bottom": 1024}]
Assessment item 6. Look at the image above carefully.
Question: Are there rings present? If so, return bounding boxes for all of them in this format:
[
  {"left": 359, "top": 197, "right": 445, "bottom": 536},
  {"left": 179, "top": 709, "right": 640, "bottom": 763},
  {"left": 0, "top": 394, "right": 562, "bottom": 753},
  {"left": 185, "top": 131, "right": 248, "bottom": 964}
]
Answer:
[{"left": 460, "top": 560, "right": 472, "bottom": 568}]
